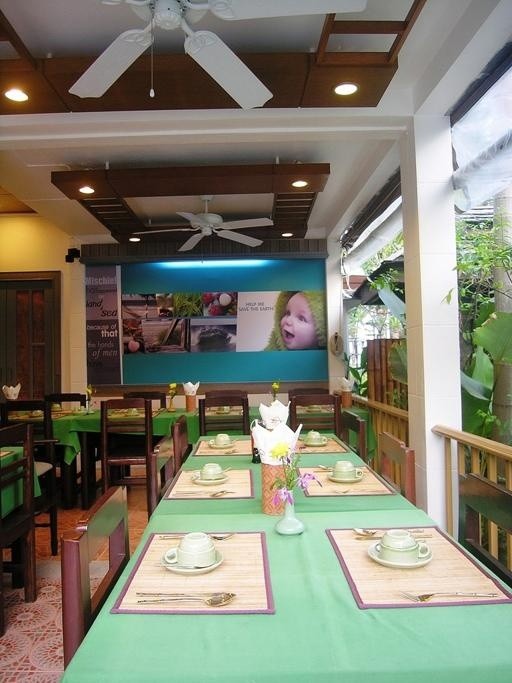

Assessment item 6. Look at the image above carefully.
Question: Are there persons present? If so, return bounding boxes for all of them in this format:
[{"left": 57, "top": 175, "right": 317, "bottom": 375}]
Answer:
[{"left": 264, "top": 289, "right": 326, "bottom": 351}]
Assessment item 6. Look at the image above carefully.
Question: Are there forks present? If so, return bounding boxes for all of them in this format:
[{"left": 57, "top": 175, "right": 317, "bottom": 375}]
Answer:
[
  {"left": 400, "top": 589, "right": 498, "bottom": 602},
  {"left": 157, "top": 529, "right": 237, "bottom": 542}
]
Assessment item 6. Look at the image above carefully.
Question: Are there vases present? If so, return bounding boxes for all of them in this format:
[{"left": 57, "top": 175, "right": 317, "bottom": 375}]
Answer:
[{"left": 276, "top": 499, "right": 304, "bottom": 537}]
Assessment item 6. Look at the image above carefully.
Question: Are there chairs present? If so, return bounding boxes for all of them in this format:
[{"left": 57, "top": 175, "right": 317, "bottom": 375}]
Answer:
[
  {"left": 1, "top": 422, "right": 36, "bottom": 636},
  {"left": 46, "top": 392, "right": 88, "bottom": 510},
  {"left": 288, "top": 395, "right": 341, "bottom": 439},
  {"left": 377, "top": 433, "right": 416, "bottom": 504},
  {"left": 62, "top": 481, "right": 130, "bottom": 669},
  {"left": 148, "top": 433, "right": 173, "bottom": 518},
  {"left": 173, "top": 414, "right": 189, "bottom": 475},
  {"left": 2, "top": 439, "right": 59, "bottom": 583},
  {"left": 205, "top": 390, "right": 249, "bottom": 396},
  {"left": 288, "top": 387, "right": 330, "bottom": 406},
  {"left": 0, "top": 401, "right": 72, "bottom": 511},
  {"left": 124, "top": 391, "right": 167, "bottom": 408},
  {"left": 100, "top": 399, "right": 166, "bottom": 503},
  {"left": 457, "top": 469, "right": 510, "bottom": 586},
  {"left": 198, "top": 396, "right": 250, "bottom": 435},
  {"left": 340, "top": 411, "right": 368, "bottom": 462}
]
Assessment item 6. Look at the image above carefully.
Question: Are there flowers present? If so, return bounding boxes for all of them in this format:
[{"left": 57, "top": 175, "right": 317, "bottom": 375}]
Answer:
[{"left": 265, "top": 441, "right": 323, "bottom": 504}]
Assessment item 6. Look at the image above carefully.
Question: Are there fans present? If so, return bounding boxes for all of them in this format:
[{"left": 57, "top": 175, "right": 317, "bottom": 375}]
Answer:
[
  {"left": 125, "top": 195, "right": 277, "bottom": 252},
  {"left": 69, "top": 0, "right": 369, "bottom": 111}
]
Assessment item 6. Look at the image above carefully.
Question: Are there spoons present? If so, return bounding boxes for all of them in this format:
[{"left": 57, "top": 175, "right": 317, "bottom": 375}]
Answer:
[
  {"left": 136, "top": 591, "right": 236, "bottom": 607},
  {"left": 172, "top": 489, "right": 228, "bottom": 498},
  {"left": 349, "top": 525, "right": 425, "bottom": 537},
  {"left": 316, "top": 463, "right": 331, "bottom": 470}
]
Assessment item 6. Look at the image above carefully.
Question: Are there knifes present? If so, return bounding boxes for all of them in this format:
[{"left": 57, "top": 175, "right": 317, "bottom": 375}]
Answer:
[
  {"left": 313, "top": 468, "right": 331, "bottom": 472},
  {"left": 352, "top": 534, "right": 432, "bottom": 542},
  {"left": 179, "top": 490, "right": 237, "bottom": 493},
  {"left": 135, "top": 589, "right": 240, "bottom": 597}
]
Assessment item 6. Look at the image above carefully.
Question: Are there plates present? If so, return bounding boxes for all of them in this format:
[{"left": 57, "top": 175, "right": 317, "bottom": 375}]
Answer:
[
  {"left": 207, "top": 444, "right": 233, "bottom": 448},
  {"left": 327, "top": 471, "right": 362, "bottom": 482},
  {"left": 161, "top": 551, "right": 222, "bottom": 574},
  {"left": 191, "top": 473, "right": 231, "bottom": 485},
  {"left": 303, "top": 441, "right": 327, "bottom": 446},
  {"left": 368, "top": 539, "right": 433, "bottom": 570}
]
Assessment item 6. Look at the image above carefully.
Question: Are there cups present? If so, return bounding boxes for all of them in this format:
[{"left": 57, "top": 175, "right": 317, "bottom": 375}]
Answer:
[
  {"left": 31, "top": 402, "right": 139, "bottom": 416},
  {"left": 305, "top": 429, "right": 327, "bottom": 442},
  {"left": 165, "top": 531, "right": 214, "bottom": 567},
  {"left": 331, "top": 460, "right": 361, "bottom": 477},
  {"left": 201, "top": 462, "right": 225, "bottom": 479},
  {"left": 378, "top": 526, "right": 428, "bottom": 562},
  {"left": 209, "top": 433, "right": 232, "bottom": 445}
]
